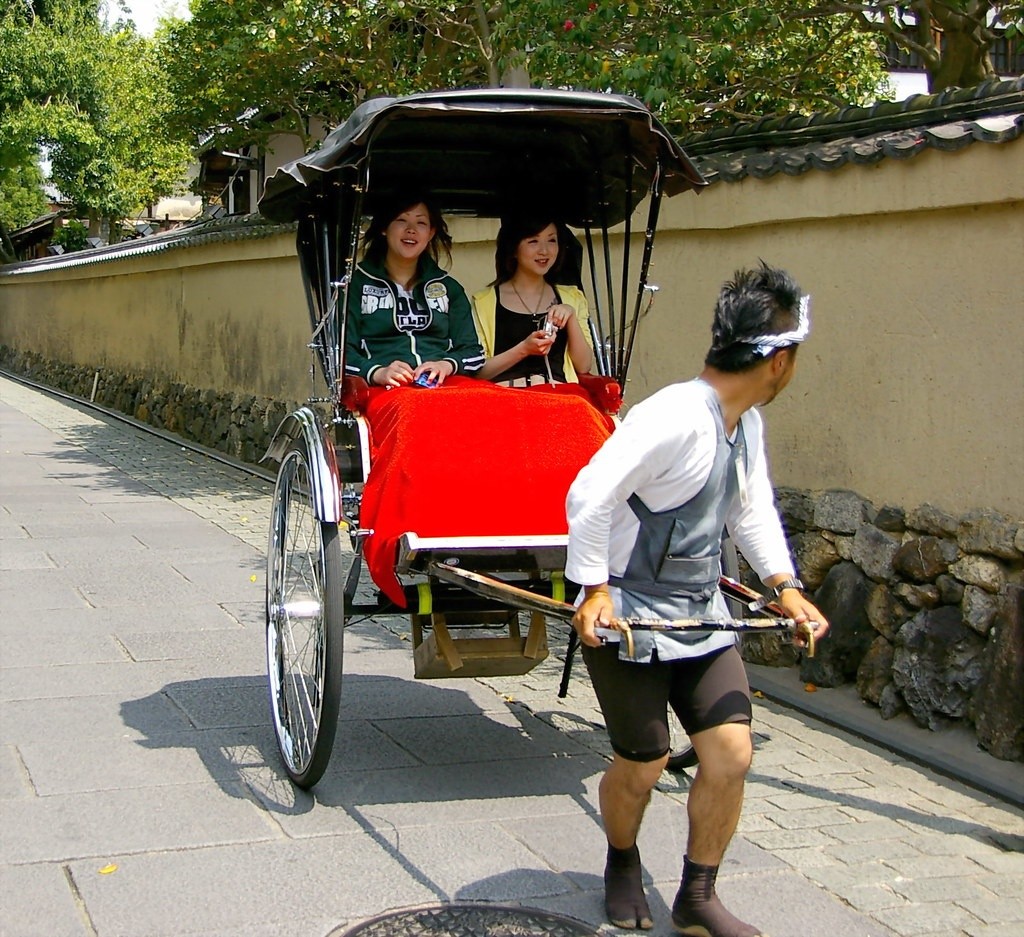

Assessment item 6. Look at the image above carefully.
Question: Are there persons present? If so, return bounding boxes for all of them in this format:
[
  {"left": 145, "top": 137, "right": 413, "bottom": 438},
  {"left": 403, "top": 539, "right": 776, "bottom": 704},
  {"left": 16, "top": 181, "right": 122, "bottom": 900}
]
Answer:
[
  {"left": 333, "top": 188, "right": 493, "bottom": 535},
  {"left": 564, "top": 259, "right": 830, "bottom": 937},
  {"left": 472, "top": 202, "right": 606, "bottom": 535}
]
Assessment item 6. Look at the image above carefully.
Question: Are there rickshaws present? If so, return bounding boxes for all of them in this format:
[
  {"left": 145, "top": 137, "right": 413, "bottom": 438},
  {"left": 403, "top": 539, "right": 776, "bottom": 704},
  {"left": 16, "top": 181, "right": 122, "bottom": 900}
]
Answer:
[{"left": 261, "top": 87, "right": 819, "bottom": 795}]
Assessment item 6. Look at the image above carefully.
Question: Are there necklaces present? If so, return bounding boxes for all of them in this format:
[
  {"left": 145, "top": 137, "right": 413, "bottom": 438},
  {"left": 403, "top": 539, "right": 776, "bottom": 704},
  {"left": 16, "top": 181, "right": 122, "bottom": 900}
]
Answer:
[{"left": 511, "top": 280, "right": 545, "bottom": 316}]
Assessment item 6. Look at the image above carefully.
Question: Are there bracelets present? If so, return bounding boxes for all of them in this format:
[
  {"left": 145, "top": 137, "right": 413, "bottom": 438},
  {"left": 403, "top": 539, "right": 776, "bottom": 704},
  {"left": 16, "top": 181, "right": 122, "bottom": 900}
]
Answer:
[{"left": 748, "top": 579, "right": 803, "bottom": 611}]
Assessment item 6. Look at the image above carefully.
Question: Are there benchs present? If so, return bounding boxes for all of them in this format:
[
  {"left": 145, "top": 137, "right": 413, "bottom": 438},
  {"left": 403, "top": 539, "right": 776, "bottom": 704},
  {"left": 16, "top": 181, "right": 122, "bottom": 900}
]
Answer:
[{"left": 336, "top": 372, "right": 621, "bottom": 497}]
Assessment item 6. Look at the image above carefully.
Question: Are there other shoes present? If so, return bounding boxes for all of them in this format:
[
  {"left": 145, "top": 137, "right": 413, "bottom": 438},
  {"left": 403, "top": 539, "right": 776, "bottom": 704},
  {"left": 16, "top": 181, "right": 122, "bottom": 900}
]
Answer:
[
  {"left": 605, "top": 842, "right": 654, "bottom": 929},
  {"left": 671, "top": 854, "right": 763, "bottom": 937}
]
